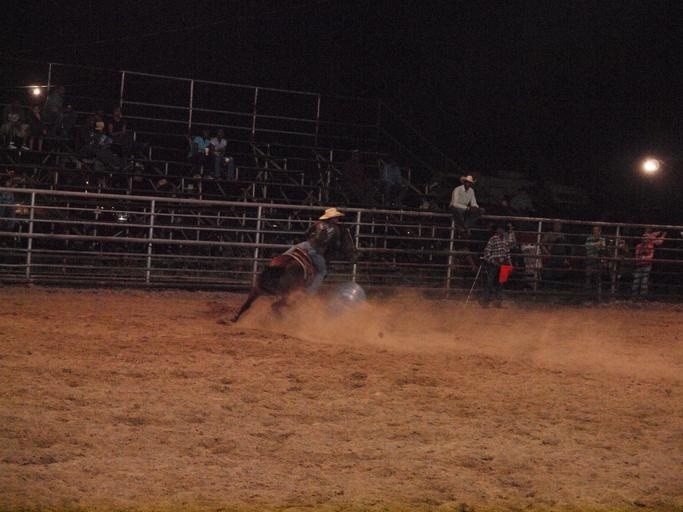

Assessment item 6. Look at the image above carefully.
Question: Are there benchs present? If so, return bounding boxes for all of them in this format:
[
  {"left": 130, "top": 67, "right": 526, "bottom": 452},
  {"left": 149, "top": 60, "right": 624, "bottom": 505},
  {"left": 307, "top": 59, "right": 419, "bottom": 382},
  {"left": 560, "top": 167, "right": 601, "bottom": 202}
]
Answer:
[{"left": 0, "top": 89, "right": 484, "bottom": 287}]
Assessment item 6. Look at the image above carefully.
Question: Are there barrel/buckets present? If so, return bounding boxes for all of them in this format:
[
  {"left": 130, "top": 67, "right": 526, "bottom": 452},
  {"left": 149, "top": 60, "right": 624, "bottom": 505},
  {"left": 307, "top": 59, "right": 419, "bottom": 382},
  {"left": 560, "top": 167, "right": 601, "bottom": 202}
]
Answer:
[{"left": 327, "top": 282, "right": 369, "bottom": 319}]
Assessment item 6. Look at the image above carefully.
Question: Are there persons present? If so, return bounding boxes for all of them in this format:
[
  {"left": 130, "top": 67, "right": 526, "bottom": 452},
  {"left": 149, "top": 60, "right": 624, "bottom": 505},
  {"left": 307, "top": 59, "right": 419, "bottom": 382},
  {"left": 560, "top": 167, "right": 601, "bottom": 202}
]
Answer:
[
  {"left": 282, "top": 208, "right": 345, "bottom": 300},
  {"left": 191, "top": 125, "right": 234, "bottom": 178},
  {"left": 1, "top": 83, "right": 128, "bottom": 244},
  {"left": 481, "top": 226, "right": 513, "bottom": 309},
  {"left": 420, "top": 175, "right": 570, "bottom": 291},
  {"left": 584, "top": 224, "right": 667, "bottom": 302},
  {"left": 382, "top": 157, "right": 409, "bottom": 205}
]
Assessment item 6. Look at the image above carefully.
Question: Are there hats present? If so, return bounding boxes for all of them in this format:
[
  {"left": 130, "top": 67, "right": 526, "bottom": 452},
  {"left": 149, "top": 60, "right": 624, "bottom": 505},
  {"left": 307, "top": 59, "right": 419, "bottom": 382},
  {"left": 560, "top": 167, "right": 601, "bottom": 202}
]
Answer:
[
  {"left": 459, "top": 175, "right": 477, "bottom": 184},
  {"left": 94, "top": 121, "right": 104, "bottom": 131},
  {"left": 318, "top": 208, "right": 344, "bottom": 221}
]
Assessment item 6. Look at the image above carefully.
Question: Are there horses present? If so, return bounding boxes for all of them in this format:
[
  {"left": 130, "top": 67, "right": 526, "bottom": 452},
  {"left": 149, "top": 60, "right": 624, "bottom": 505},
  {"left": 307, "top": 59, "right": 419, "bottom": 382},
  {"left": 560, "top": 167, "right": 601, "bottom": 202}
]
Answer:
[{"left": 229, "top": 220, "right": 360, "bottom": 323}]
